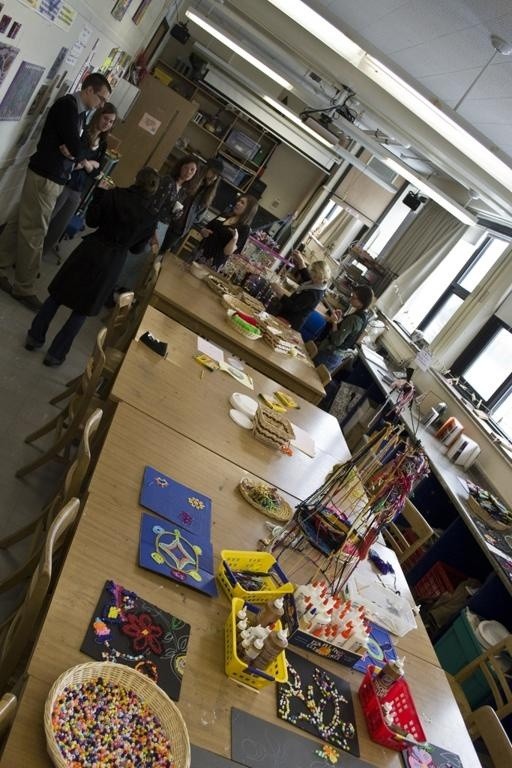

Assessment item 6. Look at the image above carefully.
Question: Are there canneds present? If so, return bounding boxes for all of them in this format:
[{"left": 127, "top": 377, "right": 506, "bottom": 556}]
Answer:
[{"left": 98, "top": 177, "right": 115, "bottom": 190}]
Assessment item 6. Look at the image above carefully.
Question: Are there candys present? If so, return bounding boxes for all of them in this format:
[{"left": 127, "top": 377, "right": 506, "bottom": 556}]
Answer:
[{"left": 49, "top": 676, "right": 177, "bottom": 768}]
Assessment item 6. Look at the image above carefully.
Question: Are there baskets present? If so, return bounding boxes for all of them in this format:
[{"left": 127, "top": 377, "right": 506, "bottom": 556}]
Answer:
[
  {"left": 216, "top": 547, "right": 294, "bottom": 597},
  {"left": 252, "top": 401, "right": 295, "bottom": 447},
  {"left": 394, "top": 525, "right": 455, "bottom": 599},
  {"left": 204, "top": 275, "right": 297, "bottom": 354},
  {"left": 468, "top": 491, "right": 509, "bottom": 531},
  {"left": 42, "top": 658, "right": 191, "bottom": 767},
  {"left": 223, "top": 594, "right": 289, "bottom": 686},
  {"left": 359, "top": 662, "right": 428, "bottom": 753}
]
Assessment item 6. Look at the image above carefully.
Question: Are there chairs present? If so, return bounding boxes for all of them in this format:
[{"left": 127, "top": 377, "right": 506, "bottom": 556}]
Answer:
[
  {"left": 175, "top": 229, "right": 204, "bottom": 257},
  {"left": 0, "top": 251, "right": 482, "bottom": 768}
]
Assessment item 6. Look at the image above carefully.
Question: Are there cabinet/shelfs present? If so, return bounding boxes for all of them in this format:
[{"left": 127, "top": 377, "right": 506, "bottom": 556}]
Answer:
[{"left": 150, "top": 57, "right": 276, "bottom": 218}]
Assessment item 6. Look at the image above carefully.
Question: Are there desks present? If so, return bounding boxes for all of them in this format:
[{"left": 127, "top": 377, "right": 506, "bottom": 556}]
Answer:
[{"left": 323, "top": 342, "right": 512, "bottom": 756}]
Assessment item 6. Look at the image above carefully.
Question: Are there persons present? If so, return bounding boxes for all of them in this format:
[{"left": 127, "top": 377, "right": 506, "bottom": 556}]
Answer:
[
  {"left": 157, "top": 158, "right": 227, "bottom": 256},
  {"left": 310, "top": 284, "right": 376, "bottom": 375},
  {"left": 21, "top": 166, "right": 162, "bottom": 369},
  {"left": 42, "top": 99, "right": 121, "bottom": 259},
  {"left": 268, "top": 248, "right": 329, "bottom": 332},
  {"left": 189, "top": 194, "right": 259, "bottom": 276},
  {"left": 1, "top": 71, "right": 114, "bottom": 312},
  {"left": 145, "top": 153, "right": 199, "bottom": 258}
]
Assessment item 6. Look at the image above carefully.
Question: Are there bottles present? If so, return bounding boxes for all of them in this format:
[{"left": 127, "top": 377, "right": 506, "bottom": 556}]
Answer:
[
  {"left": 373, "top": 656, "right": 406, "bottom": 699},
  {"left": 294, "top": 579, "right": 370, "bottom": 654},
  {"left": 236, "top": 597, "right": 289, "bottom": 671}
]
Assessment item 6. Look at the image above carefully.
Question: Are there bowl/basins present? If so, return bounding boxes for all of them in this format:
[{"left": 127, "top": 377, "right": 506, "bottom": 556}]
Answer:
[{"left": 190, "top": 260, "right": 209, "bottom": 280}]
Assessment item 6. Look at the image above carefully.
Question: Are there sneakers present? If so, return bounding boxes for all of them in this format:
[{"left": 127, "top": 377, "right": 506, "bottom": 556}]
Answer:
[{"left": 3, "top": 273, "right": 62, "bottom": 368}]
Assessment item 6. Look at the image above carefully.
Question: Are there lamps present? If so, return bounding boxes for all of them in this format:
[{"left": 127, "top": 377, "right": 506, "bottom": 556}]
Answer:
[{"left": 185, "top": 0, "right": 512, "bottom": 225}]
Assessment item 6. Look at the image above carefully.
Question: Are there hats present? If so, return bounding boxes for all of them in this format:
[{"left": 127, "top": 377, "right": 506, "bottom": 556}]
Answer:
[{"left": 207, "top": 157, "right": 225, "bottom": 178}]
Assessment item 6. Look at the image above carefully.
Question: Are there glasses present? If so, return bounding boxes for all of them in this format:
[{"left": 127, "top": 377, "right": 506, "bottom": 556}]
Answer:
[{"left": 95, "top": 93, "right": 105, "bottom": 103}]
